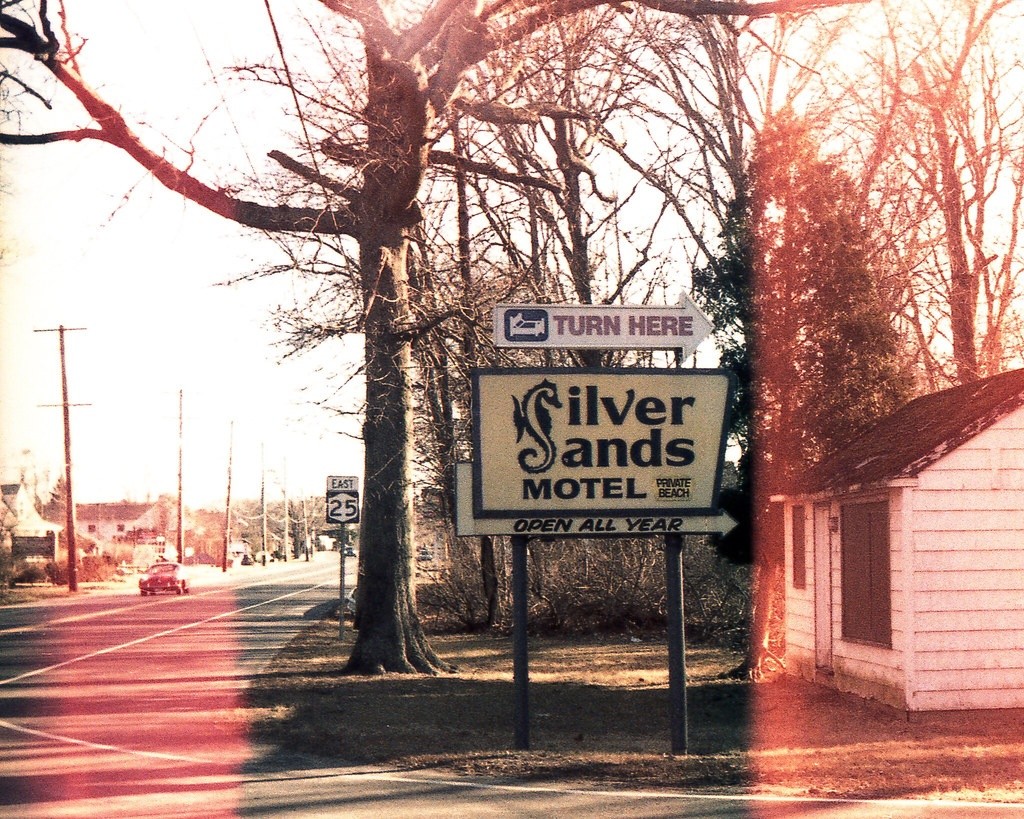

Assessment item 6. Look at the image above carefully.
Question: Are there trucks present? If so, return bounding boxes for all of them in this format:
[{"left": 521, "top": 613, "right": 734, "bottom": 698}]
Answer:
[{"left": 7, "top": 530, "right": 59, "bottom": 589}]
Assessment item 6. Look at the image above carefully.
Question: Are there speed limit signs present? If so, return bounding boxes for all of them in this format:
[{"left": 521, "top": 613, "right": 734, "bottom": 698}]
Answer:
[{"left": 326, "top": 477, "right": 361, "bottom": 524}]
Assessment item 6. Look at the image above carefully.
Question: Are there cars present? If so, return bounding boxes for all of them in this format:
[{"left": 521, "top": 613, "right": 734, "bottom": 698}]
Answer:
[
  {"left": 138, "top": 561, "right": 190, "bottom": 596},
  {"left": 344, "top": 546, "right": 356, "bottom": 557},
  {"left": 416, "top": 550, "right": 433, "bottom": 560}
]
